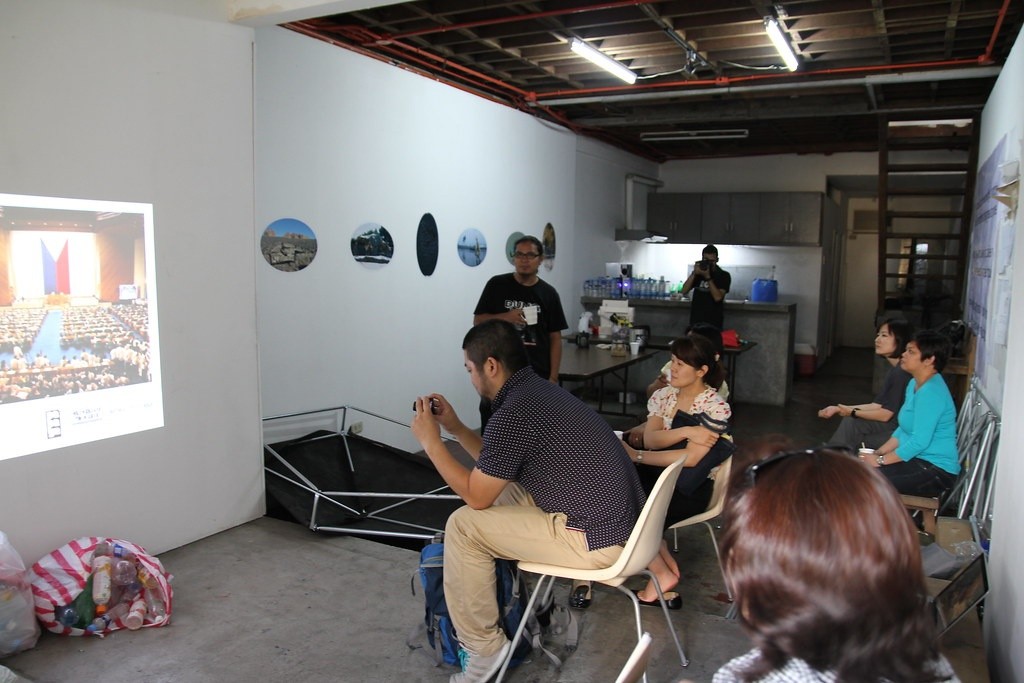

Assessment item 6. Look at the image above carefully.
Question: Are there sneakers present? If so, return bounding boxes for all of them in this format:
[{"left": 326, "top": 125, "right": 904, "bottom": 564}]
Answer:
[{"left": 449, "top": 640, "right": 511, "bottom": 683}]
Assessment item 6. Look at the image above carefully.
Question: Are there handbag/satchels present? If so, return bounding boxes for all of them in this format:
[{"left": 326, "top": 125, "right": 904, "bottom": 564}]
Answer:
[
  {"left": 28, "top": 536, "right": 173, "bottom": 637},
  {"left": 0, "top": 530, "right": 40, "bottom": 660},
  {"left": 658, "top": 409, "right": 740, "bottom": 496}
]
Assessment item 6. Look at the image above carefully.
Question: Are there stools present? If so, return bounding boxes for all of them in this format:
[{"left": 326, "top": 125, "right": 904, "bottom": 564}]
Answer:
[{"left": 900, "top": 493, "right": 940, "bottom": 537}]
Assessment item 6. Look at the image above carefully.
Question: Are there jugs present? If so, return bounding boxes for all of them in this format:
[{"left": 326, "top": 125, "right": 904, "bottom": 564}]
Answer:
[
  {"left": 575, "top": 330, "right": 590, "bottom": 349},
  {"left": 629, "top": 325, "right": 651, "bottom": 350}
]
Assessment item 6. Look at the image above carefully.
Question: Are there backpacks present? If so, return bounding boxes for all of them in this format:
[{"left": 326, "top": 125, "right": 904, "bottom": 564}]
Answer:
[{"left": 410, "top": 531, "right": 541, "bottom": 666}]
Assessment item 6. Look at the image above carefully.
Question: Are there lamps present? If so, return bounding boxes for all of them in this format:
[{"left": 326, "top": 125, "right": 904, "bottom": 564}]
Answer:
[
  {"left": 639, "top": 128, "right": 749, "bottom": 141},
  {"left": 566, "top": 37, "right": 637, "bottom": 86},
  {"left": 763, "top": 15, "right": 800, "bottom": 72}
]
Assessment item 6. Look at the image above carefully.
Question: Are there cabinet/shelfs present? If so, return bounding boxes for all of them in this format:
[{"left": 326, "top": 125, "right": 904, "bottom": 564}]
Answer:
[{"left": 647, "top": 191, "right": 826, "bottom": 247}]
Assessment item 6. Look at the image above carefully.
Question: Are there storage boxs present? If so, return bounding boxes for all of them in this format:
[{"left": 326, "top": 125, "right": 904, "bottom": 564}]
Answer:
[
  {"left": 934, "top": 516, "right": 974, "bottom": 558},
  {"left": 597, "top": 299, "right": 636, "bottom": 336},
  {"left": 795, "top": 342, "right": 818, "bottom": 377}
]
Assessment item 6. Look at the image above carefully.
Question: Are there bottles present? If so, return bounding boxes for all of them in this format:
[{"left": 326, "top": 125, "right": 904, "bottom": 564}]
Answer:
[
  {"left": 0, "top": 601, "right": 23, "bottom": 654},
  {"left": 611, "top": 339, "right": 627, "bottom": 356},
  {"left": 53, "top": 540, "right": 166, "bottom": 633},
  {"left": 583, "top": 275, "right": 684, "bottom": 300}
]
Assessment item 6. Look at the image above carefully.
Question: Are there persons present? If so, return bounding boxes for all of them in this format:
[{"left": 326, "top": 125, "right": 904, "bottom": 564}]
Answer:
[
  {"left": 619, "top": 334, "right": 732, "bottom": 609},
  {"left": 411, "top": 317, "right": 646, "bottom": 682},
  {"left": 472, "top": 235, "right": 569, "bottom": 436},
  {"left": 680, "top": 244, "right": 731, "bottom": 361},
  {"left": 816, "top": 315, "right": 963, "bottom": 498},
  {"left": 0, "top": 290, "right": 152, "bottom": 405},
  {"left": 708, "top": 449, "right": 963, "bottom": 682},
  {"left": 646, "top": 323, "right": 730, "bottom": 401}
]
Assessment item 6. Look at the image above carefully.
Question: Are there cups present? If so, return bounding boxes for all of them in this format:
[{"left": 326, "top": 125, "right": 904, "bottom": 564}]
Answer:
[
  {"left": 613, "top": 431, "right": 623, "bottom": 440},
  {"left": 859, "top": 447, "right": 874, "bottom": 462},
  {"left": 519, "top": 306, "right": 537, "bottom": 325},
  {"left": 630, "top": 342, "right": 639, "bottom": 355}
]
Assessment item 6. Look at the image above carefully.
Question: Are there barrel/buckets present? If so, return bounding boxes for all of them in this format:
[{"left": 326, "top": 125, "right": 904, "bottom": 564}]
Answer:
[{"left": 751, "top": 278, "right": 778, "bottom": 303}]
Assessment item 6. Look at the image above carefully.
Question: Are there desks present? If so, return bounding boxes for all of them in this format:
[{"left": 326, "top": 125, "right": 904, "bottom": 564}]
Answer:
[
  {"left": 561, "top": 333, "right": 758, "bottom": 406},
  {"left": 556, "top": 342, "right": 662, "bottom": 419}
]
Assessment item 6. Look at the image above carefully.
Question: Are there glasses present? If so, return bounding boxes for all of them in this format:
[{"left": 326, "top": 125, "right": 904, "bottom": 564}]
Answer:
[
  {"left": 514, "top": 252, "right": 539, "bottom": 259},
  {"left": 751, "top": 447, "right": 854, "bottom": 487}
]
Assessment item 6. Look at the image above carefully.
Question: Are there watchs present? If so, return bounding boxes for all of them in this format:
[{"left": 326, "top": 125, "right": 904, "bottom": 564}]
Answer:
[{"left": 877, "top": 456, "right": 885, "bottom": 466}]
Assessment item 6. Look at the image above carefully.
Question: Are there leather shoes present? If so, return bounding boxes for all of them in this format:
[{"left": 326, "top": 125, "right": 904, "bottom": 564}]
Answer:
[
  {"left": 625, "top": 589, "right": 682, "bottom": 610},
  {"left": 569, "top": 585, "right": 592, "bottom": 610}
]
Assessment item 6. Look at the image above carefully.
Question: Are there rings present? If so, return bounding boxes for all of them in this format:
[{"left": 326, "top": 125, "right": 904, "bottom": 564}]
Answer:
[{"left": 636, "top": 437, "right": 638, "bottom": 441}]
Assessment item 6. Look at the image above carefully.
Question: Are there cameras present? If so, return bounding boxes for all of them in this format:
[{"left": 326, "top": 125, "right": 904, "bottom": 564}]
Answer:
[
  {"left": 699, "top": 260, "right": 712, "bottom": 270},
  {"left": 413, "top": 398, "right": 434, "bottom": 414}
]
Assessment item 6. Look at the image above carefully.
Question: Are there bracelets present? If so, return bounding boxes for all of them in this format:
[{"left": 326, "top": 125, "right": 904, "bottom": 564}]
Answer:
[
  {"left": 851, "top": 408, "right": 861, "bottom": 418},
  {"left": 706, "top": 277, "right": 712, "bottom": 281},
  {"left": 636, "top": 450, "right": 643, "bottom": 463}
]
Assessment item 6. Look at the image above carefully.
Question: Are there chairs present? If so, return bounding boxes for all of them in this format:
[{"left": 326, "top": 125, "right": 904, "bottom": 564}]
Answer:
[
  {"left": 662, "top": 427, "right": 740, "bottom": 604},
  {"left": 492, "top": 451, "right": 691, "bottom": 683}
]
